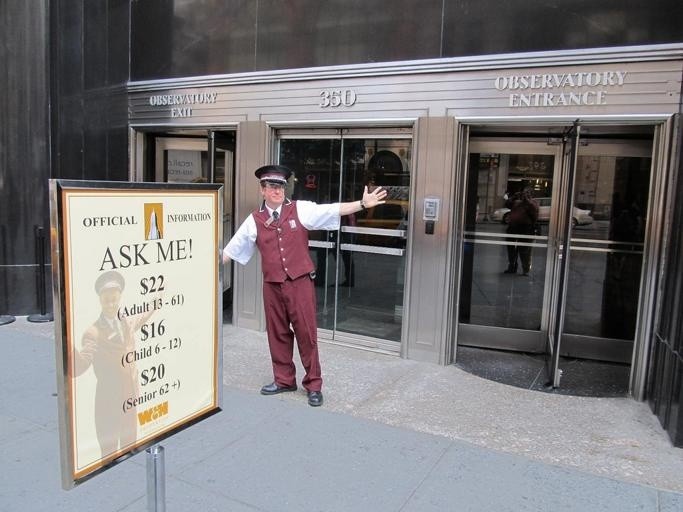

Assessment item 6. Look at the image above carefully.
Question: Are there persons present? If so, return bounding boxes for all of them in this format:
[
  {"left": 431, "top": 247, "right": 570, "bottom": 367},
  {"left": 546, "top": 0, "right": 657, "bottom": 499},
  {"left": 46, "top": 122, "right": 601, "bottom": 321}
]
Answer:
[
  {"left": 220, "top": 166, "right": 389, "bottom": 406},
  {"left": 502, "top": 186, "right": 539, "bottom": 277},
  {"left": 73, "top": 269, "right": 167, "bottom": 464},
  {"left": 307, "top": 196, "right": 356, "bottom": 288}
]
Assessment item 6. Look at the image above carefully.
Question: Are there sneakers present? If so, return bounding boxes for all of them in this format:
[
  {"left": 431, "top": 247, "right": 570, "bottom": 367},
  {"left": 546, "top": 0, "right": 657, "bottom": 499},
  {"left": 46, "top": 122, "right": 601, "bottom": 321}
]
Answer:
[
  {"left": 503, "top": 268, "right": 531, "bottom": 276},
  {"left": 311, "top": 275, "right": 358, "bottom": 290}
]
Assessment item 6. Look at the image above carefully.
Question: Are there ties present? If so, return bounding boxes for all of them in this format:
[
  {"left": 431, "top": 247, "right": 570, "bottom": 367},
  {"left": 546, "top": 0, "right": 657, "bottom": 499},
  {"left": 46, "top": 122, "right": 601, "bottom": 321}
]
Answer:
[{"left": 272, "top": 211, "right": 278, "bottom": 224}]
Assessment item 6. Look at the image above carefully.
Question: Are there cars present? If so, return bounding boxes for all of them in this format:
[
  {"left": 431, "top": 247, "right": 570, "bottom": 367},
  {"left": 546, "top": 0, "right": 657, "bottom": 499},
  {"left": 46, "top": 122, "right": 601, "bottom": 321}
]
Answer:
[
  {"left": 490, "top": 197, "right": 593, "bottom": 230},
  {"left": 309, "top": 200, "right": 408, "bottom": 251}
]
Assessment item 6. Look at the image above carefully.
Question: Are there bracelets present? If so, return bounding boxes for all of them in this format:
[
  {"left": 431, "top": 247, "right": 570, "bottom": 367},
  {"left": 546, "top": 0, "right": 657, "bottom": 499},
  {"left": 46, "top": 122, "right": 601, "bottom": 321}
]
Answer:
[{"left": 359, "top": 200, "right": 366, "bottom": 210}]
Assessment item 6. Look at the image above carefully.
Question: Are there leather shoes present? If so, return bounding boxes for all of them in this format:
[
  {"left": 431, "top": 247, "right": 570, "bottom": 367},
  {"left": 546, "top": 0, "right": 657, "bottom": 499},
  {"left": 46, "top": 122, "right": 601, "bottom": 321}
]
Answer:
[
  {"left": 260, "top": 382, "right": 297, "bottom": 395},
  {"left": 307, "top": 389, "right": 323, "bottom": 406}
]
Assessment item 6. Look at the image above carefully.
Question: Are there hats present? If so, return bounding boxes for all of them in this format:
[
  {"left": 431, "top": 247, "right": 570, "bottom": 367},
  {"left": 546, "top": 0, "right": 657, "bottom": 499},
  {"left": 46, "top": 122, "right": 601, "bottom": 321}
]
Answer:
[
  {"left": 254, "top": 165, "right": 291, "bottom": 186},
  {"left": 94, "top": 270, "right": 124, "bottom": 294}
]
